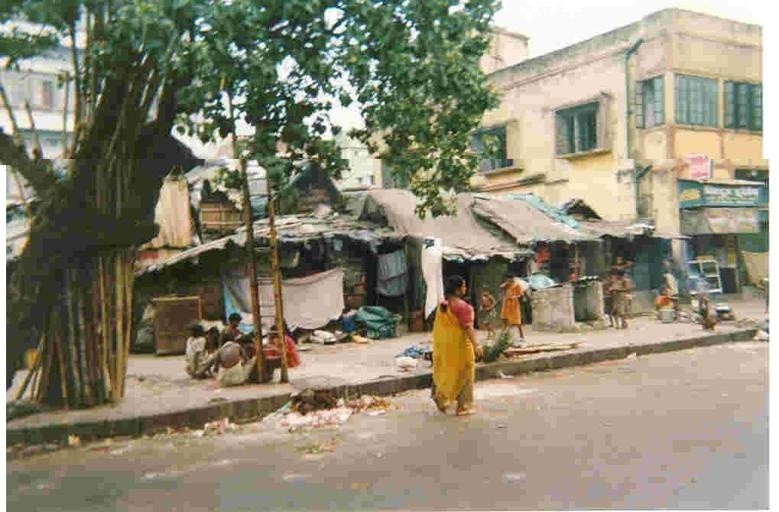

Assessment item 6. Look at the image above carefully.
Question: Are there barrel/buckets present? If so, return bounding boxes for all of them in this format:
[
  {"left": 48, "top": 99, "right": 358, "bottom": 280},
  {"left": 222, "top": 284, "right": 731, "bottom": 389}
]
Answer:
[{"left": 659, "top": 308, "right": 676, "bottom": 324}]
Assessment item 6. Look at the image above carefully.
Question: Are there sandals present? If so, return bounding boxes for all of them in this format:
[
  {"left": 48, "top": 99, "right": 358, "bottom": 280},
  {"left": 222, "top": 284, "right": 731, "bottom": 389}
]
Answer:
[
  {"left": 432, "top": 399, "right": 446, "bottom": 415},
  {"left": 456, "top": 408, "right": 474, "bottom": 415}
]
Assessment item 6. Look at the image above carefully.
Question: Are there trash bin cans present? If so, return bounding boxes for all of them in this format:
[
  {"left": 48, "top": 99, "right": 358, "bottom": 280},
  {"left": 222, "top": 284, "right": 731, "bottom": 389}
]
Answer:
[{"left": 573, "top": 275, "right": 605, "bottom": 322}]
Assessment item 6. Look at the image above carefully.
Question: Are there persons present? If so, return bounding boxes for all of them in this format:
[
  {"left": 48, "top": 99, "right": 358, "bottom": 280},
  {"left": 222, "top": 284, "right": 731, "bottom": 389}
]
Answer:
[
  {"left": 662, "top": 265, "right": 679, "bottom": 299},
  {"left": 478, "top": 288, "right": 497, "bottom": 337},
  {"left": 499, "top": 274, "right": 525, "bottom": 338},
  {"left": 430, "top": 274, "right": 483, "bottom": 416},
  {"left": 606, "top": 270, "right": 619, "bottom": 326},
  {"left": 612, "top": 271, "right": 634, "bottom": 329},
  {"left": 183, "top": 312, "right": 302, "bottom": 386}
]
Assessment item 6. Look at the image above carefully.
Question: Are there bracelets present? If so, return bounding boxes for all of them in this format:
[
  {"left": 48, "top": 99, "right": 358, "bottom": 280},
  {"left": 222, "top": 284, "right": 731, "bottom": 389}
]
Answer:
[{"left": 473, "top": 345, "right": 480, "bottom": 351}]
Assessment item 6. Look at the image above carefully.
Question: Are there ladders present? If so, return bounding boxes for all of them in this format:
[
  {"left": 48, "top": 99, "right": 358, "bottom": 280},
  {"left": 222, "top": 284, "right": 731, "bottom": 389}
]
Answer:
[{"left": 228, "top": 86, "right": 289, "bottom": 383}]
